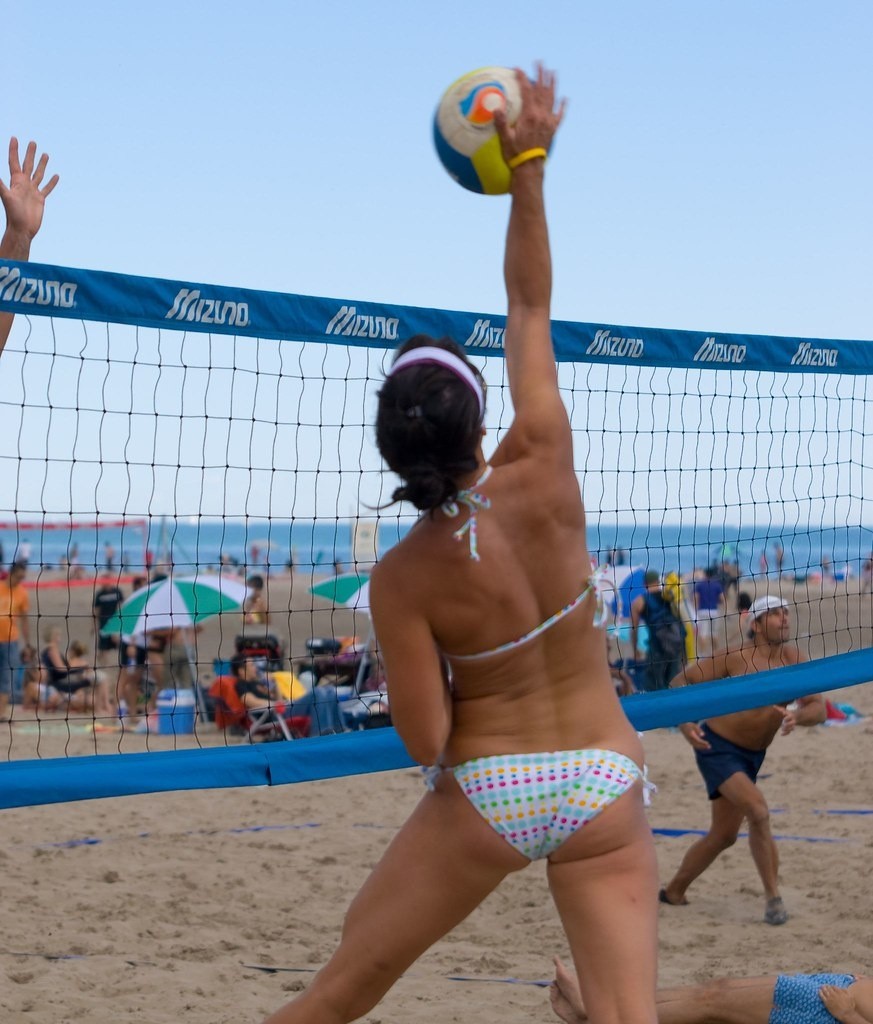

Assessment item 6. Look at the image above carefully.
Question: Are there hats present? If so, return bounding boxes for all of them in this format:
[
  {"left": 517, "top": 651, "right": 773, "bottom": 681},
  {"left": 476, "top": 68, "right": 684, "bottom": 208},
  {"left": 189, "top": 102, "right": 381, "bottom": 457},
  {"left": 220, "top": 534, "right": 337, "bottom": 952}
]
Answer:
[{"left": 745, "top": 595, "right": 789, "bottom": 638}]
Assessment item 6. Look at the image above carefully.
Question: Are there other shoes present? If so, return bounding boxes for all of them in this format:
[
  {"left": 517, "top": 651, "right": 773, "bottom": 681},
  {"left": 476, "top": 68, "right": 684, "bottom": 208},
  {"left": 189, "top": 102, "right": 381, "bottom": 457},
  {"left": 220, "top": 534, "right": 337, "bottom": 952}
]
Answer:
[{"left": 765, "top": 895, "right": 788, "bottom": 926}]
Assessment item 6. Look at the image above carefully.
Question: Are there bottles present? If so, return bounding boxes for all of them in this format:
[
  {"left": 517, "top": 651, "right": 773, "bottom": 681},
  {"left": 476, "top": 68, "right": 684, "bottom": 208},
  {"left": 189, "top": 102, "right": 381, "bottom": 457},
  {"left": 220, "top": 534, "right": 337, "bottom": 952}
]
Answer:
[
  {"left": 125, "top": 658, "right": 136, "bottom": 676},
  {"left": 117, "top": 696, "right": 127, "bottom": 727}
]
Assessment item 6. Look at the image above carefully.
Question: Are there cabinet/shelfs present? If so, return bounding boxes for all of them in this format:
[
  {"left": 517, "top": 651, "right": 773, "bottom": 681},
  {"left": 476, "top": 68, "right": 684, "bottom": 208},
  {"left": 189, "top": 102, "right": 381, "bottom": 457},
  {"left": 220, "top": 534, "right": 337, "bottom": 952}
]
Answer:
[{"left": 156, "top": 689, "right": 196, "bottom": 734}]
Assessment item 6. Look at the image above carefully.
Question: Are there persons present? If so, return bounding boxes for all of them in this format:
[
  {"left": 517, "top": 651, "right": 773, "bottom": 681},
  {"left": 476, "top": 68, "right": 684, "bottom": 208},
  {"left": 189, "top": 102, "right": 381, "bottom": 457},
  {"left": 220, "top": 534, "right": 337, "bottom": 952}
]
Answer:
[
  {"left": 660, "top": 594, "right": 826, "bottom": 927},
  {"left": 260, "top": 61, "right": 665, "bottom": 1024},
  {"left": 592, "top": 537, "right": 873, "bottom": 696},
  {"left": 0, "top": 135, "right": 60, "bottom": 359},
  {"left": 546, "top": 955, "right": 873, "bottom": 1024},
  {"left": 1, "top": 537, "right": 389, "bottom": 744}
]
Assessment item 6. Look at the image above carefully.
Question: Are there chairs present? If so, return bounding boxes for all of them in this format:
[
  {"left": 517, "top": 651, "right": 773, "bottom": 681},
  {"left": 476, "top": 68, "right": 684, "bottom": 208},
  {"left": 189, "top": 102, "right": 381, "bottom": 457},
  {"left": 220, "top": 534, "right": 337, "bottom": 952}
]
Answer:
[{"left": 208, "top": 676, "right": 311, "bottom": 744}]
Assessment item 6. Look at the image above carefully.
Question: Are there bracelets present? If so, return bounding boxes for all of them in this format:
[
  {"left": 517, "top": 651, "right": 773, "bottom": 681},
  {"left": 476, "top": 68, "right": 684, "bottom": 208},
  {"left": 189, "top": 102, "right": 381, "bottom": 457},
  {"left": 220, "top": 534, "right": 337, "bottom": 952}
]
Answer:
[{"left": 509, "top": 146, "right": 547, "bottom": 171}]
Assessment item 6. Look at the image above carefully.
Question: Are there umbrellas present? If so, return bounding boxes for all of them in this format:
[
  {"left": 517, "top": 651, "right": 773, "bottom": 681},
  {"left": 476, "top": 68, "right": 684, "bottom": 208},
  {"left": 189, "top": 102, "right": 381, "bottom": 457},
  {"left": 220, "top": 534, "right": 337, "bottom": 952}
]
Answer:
[
  {"left": 307, "top": 573, "right": 376, "bottom": 697},
  {"left": 98, "top": 574, "right": 252, "bottom": 733}
]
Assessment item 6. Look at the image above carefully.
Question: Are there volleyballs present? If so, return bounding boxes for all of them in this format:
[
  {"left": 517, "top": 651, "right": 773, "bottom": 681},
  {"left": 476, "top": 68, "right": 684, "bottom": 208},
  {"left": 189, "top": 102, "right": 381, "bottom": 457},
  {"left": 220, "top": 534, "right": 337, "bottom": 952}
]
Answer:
[{"left": 430, "top": 66, "right": 550, "bottom": 199}]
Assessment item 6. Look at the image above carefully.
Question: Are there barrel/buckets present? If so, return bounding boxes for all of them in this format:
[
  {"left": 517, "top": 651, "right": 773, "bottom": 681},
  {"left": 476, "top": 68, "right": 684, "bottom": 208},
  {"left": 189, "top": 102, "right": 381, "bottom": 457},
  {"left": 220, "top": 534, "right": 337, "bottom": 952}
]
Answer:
[{"left": 156, "top": 689, "right": 196, "bottom": 735}]
